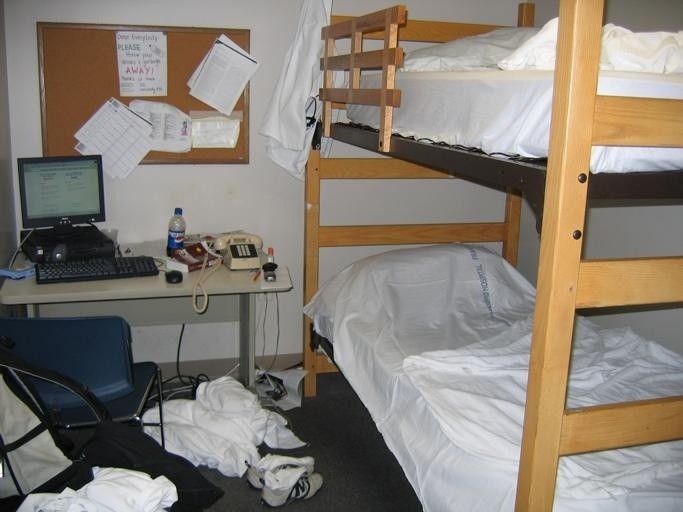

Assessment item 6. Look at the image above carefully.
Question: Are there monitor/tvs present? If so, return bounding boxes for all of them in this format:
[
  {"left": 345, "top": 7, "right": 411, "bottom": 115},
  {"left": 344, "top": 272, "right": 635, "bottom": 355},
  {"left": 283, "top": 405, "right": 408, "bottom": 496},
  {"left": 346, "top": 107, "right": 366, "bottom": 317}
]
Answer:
[{"left": 16, "top": 154, "right": 106, "bottom": 240}]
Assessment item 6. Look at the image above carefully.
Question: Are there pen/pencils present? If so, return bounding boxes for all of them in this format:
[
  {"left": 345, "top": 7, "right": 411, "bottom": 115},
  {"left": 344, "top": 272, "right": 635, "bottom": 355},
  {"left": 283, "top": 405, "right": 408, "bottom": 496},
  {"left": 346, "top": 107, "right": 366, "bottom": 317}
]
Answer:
[{"left": 253, "top": 271, "right": 261, "bottom": 281}]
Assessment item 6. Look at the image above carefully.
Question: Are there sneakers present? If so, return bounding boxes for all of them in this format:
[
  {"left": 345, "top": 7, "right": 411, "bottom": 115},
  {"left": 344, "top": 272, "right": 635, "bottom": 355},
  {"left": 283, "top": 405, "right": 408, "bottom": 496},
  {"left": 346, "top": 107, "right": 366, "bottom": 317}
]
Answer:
[
  {"left": 245, "top": 454, "right": 315, "bottom": 490},
  {"left": 260, "top": 473, "right": 323, "bottom": 507}
]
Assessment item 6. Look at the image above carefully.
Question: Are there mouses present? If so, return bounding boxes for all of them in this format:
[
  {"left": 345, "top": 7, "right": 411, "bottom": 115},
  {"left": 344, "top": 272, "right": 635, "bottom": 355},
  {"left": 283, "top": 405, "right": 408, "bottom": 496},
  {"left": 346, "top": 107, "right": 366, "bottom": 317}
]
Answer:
[{"left": 165, "top": 270, "right": 183, "bottom": 284}]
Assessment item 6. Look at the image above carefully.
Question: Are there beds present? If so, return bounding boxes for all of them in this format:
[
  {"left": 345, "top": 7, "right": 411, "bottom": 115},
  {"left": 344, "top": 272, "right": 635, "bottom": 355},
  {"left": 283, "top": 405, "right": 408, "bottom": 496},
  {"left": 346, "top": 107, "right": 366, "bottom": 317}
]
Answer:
[{"left": 302, "top": 0, "right": 683, "bottom": 512}]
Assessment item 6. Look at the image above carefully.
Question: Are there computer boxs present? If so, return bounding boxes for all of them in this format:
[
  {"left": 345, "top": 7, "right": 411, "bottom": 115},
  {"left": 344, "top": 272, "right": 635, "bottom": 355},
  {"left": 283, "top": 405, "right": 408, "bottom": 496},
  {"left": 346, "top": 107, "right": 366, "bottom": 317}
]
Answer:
[{"left": 20, "top": 225, "right": 115, "bottom": 263}]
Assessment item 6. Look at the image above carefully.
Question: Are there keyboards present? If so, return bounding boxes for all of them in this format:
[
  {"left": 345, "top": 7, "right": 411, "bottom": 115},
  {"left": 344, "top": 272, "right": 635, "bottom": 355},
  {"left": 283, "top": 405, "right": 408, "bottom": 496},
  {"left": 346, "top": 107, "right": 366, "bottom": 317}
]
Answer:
[{"left": 34, "top": 255, "right": 160, "bottom": 285}]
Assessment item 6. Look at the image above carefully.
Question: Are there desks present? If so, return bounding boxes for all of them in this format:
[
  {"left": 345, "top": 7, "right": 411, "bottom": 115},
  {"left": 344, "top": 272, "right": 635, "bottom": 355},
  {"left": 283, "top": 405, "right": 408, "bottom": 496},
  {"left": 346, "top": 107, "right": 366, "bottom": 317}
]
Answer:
[{"left": 0, "top": 240, "right": 293, "bottom": 386}]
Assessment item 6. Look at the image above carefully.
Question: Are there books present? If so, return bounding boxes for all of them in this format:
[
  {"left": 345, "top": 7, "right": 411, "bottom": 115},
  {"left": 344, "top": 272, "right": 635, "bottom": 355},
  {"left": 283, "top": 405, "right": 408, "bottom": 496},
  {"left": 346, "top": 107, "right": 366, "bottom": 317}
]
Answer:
[{"left": 167, "top": 241, "right": 224, "bottom": 273}]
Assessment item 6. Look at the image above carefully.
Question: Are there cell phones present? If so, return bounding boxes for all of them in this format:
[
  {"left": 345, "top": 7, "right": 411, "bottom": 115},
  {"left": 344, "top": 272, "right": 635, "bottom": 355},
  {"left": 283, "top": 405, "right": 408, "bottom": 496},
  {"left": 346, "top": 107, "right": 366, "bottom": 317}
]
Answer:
[{"left": 264, "top": 270, "right": 276, "bottom": 282}]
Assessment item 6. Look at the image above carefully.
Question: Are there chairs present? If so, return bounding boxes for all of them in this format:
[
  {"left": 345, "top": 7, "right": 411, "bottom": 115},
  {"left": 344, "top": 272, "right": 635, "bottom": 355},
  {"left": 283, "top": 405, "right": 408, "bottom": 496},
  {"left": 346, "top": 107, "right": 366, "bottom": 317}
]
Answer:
[{"left": 0, "top": 317, "right": 164, "bottom": 448}]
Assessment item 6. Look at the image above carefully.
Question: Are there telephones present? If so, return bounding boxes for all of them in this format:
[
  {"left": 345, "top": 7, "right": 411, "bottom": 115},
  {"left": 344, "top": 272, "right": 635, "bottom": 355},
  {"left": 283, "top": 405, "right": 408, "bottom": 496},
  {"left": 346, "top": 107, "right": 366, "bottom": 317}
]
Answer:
[{"left": 214, "top": 233, "right": 263, "bottom": 270}]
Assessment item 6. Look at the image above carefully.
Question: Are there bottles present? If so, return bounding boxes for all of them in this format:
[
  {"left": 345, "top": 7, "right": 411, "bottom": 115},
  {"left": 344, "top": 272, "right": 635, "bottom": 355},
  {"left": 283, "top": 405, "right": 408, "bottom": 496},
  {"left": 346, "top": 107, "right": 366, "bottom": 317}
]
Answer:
[
  {"left": 266, "top": 247, "right": 274, "bottom": 264},
  {"left": 167, "top": 207, "right": 187, "bottom": 258}
]
